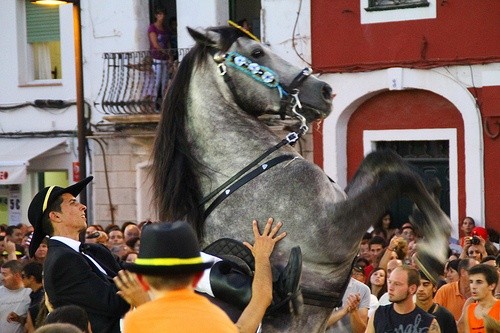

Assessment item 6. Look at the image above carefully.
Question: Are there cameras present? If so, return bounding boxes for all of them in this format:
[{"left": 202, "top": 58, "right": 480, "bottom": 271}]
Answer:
[{"left": 470, "top": 237, "right": 480, "bottom": 245}]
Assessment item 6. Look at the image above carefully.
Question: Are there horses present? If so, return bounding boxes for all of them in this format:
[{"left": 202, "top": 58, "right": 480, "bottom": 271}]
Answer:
[{"left": 148, "top": 26, "right": 452, "bottom": 333}]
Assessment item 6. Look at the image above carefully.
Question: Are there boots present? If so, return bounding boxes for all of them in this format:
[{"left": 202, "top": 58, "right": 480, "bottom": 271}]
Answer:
[{"left": 209, "top": 246, "right": 305, "bottom": 316}]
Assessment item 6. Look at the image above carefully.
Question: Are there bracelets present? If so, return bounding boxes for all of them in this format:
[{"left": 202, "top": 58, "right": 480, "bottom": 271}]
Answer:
[{"left": 388, "top": 248, "right": 394, "bottom": 251}]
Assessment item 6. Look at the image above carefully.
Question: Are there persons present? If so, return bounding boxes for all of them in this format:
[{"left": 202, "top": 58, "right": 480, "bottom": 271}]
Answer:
[
  {"left": 352, "top": 214, "right": 500, "bottom": 333},
  {"left": 45, "top": 305, "right": 92, "bottom": 333},
  {"left": 87, "top": 221, "right": 152, "bottom": 263},
  {"left": 126, "top": 56, "right": 154, "bottom": 111},
  {"left": 0, "top": 223, "right": 49, "bottom": 333},
  {"left": 113, "top": 218, "right": 286, "bottom": 333},
  {"left": 28, "top": 175, "right": 132, "bottom": 333},
  {"left": 122, "top": 220, "right": 240, "bottom": 333},
  {"left": 465, "top": 264, "right": 500, "bottom": 333},
  {"left": 34, "top": 323, "right": 82, "bottom": 333},
  {"left": 148, "top": 9, "right": 178, "bottom": 113},
  {"left": 415, "top": 270, "right": 458, "bottom": 333},
  {"left": 326, "top": 277, "right": 370, "bottom": 333},
  {"left": 236, "top": 19, "right": 252, "bottom": 34},
  {"left": 365, "top": 265, "right": 441, "bottom": 333}
]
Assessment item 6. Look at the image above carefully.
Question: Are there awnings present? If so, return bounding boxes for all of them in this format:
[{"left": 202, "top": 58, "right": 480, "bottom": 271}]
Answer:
[{"left": 0, "top": 140, "right": 65, "bottom": 185}]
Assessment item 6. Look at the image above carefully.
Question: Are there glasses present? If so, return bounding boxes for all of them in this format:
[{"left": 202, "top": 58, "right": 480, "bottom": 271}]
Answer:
[{"left": 21, "top": 274, "right": 27, "bottom": 279}]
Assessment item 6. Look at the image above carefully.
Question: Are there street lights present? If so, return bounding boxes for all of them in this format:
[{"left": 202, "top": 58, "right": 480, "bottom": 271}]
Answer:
[{"left": 29, "top": 0, "right": 88, "bottom": 246}]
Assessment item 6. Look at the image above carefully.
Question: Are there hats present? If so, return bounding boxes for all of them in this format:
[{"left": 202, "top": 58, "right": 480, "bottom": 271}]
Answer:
[
  {"left": 121, "top": 220, "right": 223, "bottom": 273},
  {"left": 352, "top": 263, "right": 364, "bottom": 273},
  {"left": 28, "top": 176, "right": 94, "bottom": 257},
  {"left": 401, "top": 223, "right": 414, "bottom": 231},
  {"left": 470, "top": 226, "right": 488, "bottom": 240}
]
